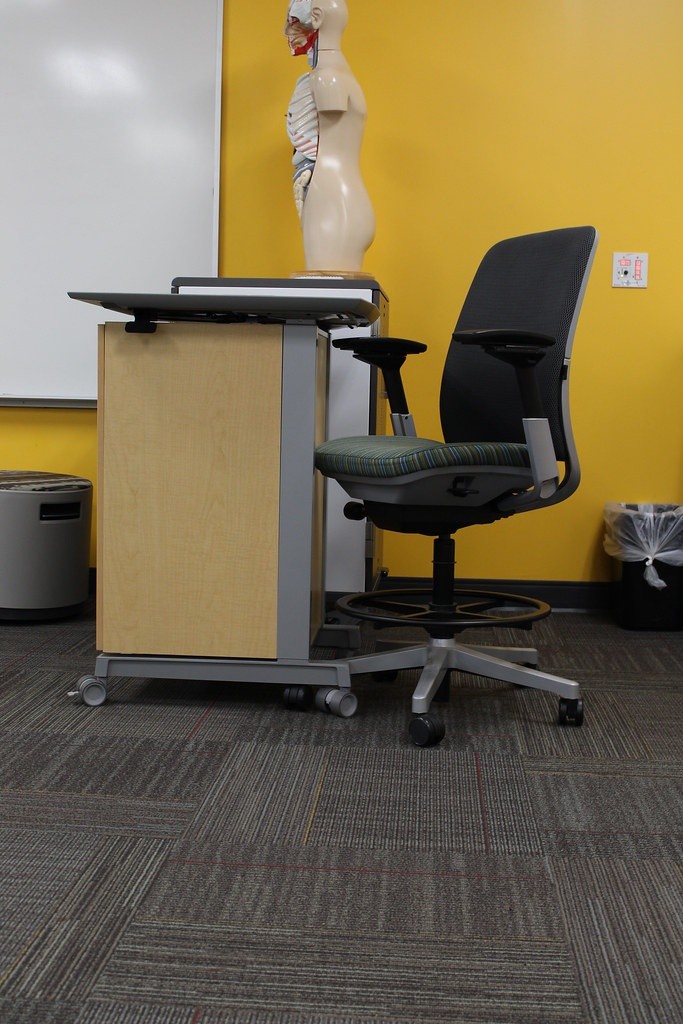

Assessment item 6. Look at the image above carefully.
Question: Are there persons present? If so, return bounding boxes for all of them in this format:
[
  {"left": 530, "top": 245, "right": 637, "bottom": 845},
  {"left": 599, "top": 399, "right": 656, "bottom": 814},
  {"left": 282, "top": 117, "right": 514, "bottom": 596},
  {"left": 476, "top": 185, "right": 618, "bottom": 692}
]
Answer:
[{"left": 282, "top": 0, "right": 375, "bottom": 274}]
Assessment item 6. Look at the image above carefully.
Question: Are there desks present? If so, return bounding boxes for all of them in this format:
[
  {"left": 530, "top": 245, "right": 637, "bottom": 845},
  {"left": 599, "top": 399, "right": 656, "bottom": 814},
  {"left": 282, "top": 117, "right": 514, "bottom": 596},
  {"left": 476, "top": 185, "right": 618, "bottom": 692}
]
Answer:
[{"left": 63, "top": 290, "right": 381, "bottom": 713}]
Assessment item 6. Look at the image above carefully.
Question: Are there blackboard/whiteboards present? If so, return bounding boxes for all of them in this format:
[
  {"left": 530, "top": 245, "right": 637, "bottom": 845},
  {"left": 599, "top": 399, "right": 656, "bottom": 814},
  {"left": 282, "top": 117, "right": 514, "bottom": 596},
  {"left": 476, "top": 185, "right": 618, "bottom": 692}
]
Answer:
[{"left": 0, "top": 0, "right": 227, "bottom": 409}]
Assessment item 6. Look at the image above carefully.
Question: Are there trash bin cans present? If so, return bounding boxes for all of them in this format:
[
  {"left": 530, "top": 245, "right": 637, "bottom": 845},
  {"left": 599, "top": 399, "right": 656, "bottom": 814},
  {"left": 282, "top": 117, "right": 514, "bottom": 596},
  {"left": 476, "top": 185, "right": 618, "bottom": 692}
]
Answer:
[{"left": 604, "top": 501, "right": 683, "bottom": 634}]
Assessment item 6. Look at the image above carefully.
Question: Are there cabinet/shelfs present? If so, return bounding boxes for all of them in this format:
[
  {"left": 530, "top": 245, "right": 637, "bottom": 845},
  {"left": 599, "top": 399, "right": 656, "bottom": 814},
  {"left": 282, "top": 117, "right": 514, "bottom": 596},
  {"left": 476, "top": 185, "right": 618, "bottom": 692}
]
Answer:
[{"left": 172, "top": 276, "right": 391, "bottom": 607}]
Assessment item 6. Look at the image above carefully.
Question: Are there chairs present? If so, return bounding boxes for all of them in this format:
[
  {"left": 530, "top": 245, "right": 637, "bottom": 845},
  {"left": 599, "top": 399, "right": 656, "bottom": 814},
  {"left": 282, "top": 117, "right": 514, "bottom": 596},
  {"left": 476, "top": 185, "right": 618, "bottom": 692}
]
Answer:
[{"left": 311, "top": 224, "right": 601, "bottom": 749}]
